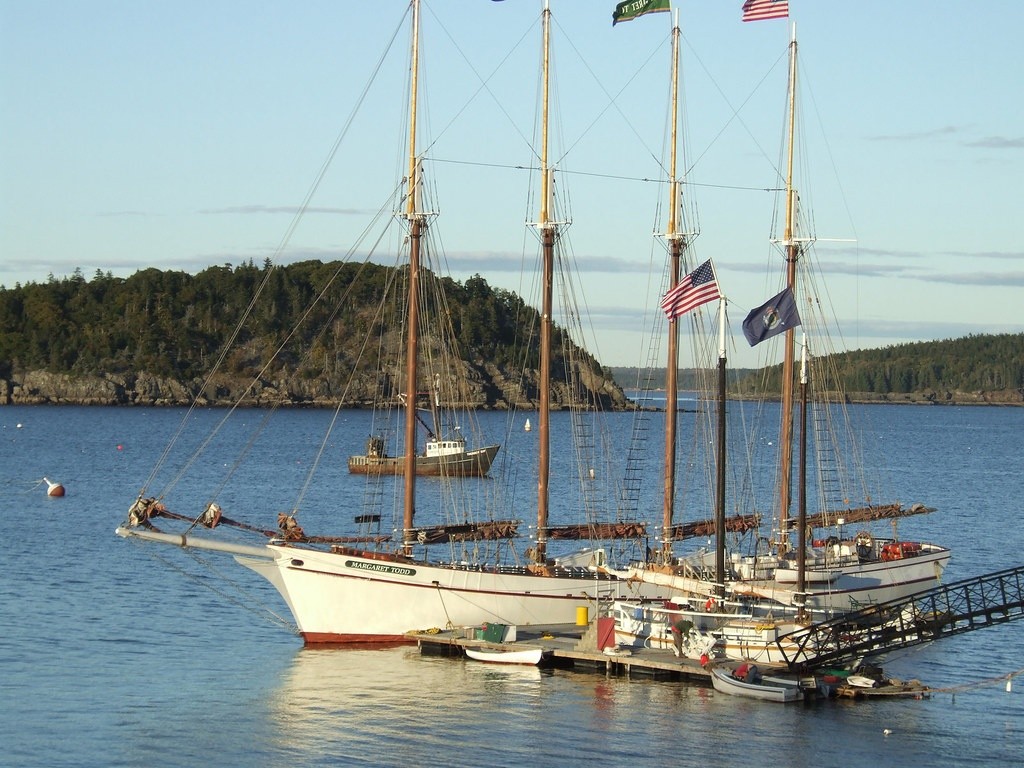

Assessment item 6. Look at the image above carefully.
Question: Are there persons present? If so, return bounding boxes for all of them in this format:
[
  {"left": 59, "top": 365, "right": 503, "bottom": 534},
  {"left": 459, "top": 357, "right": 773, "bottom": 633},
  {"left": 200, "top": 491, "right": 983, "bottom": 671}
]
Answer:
[
  {"left": 377, "top": 435, "right": 384, "bottom": 458},
  {"left": 732, "top": 663, "right": 758, "bottom": 684},
  {"left": 671, "top": 620, "right": 694, "bottom": 659}
]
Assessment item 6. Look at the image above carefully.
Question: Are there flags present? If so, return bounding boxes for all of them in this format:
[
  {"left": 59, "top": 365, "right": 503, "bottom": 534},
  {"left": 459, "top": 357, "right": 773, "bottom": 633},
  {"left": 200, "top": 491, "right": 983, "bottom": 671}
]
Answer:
[
  {"left": 742, "top": 0, "right": 789, "bottom": 22},
  {"left": 660, "top": 259, "right": 721, "bottom": 323},
  {"left": 612, "top": 0, "right": 673, "bottom": 27},
  {"left": 742, "top": 284, "right": 802, "bottom": 347}
]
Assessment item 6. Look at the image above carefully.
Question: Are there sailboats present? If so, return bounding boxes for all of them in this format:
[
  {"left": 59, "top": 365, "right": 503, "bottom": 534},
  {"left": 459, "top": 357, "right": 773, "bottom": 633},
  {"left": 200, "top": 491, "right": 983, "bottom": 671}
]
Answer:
[{"left": 113, "top": 0, "right": 955, "bottom": 646}]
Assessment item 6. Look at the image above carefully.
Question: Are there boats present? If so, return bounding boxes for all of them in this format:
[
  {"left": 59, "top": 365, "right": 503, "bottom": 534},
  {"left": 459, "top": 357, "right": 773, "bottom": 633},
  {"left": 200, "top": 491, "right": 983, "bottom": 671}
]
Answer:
[
  {"left": 466, "top": 650, "right": 555, "bottom": 666},
  {"left": 348, "top": 392, "right": 500, "bottom": 478},
  {"left": 709, "top": 666, "right": 824, "bottom": 702}
]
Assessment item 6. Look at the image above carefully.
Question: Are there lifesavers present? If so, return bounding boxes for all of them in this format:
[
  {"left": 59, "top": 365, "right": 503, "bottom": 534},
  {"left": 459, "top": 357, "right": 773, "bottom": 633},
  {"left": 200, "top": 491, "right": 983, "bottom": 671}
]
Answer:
[
  {"left": 881, "top": 541, "right": 922, "bottom": 560},
  {"left": 706, "top": 598, "right": 718, "bottom": 613}
]
